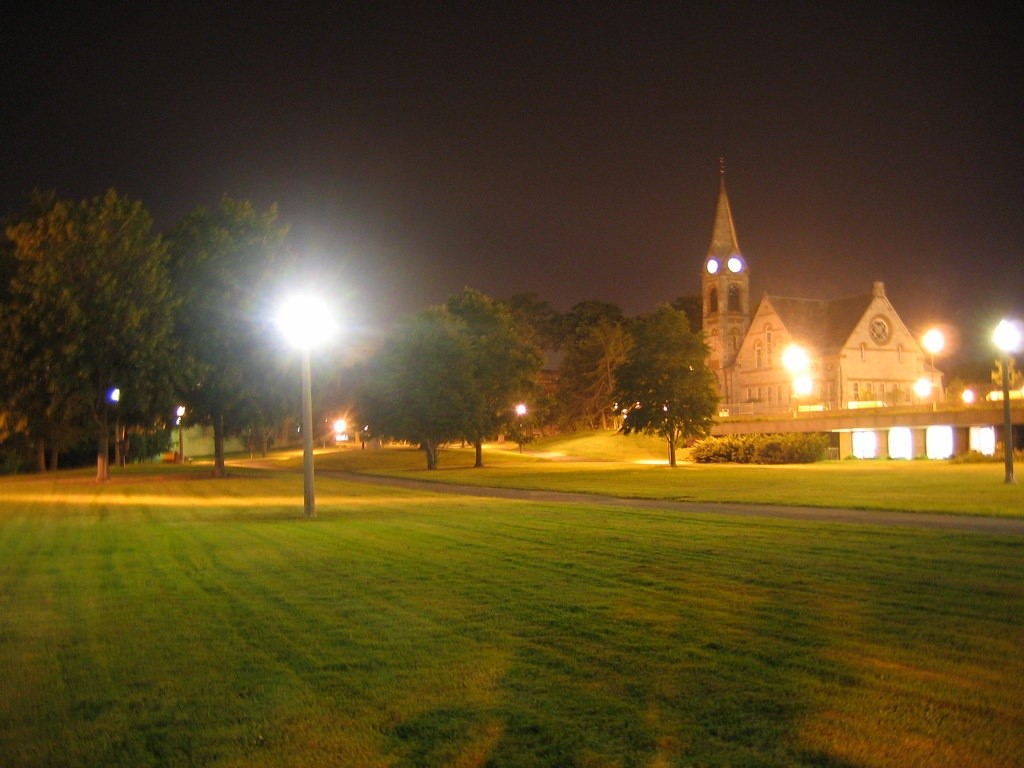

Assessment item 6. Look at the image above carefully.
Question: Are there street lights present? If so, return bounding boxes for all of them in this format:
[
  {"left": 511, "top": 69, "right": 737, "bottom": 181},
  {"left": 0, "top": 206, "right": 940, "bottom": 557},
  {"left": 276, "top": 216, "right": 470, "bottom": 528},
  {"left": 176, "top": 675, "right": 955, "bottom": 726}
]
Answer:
[
  {"left": 928, "top": 332, "right": 938, "bottom": 411},
  {"left": 292, "top": 301, "right": 317, "bottom": 517},
  {"left": 993, "top": 320, "right": 1018, "bottom": 484}
]
